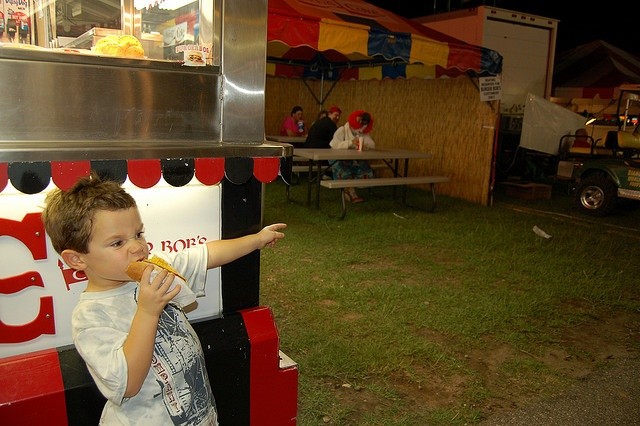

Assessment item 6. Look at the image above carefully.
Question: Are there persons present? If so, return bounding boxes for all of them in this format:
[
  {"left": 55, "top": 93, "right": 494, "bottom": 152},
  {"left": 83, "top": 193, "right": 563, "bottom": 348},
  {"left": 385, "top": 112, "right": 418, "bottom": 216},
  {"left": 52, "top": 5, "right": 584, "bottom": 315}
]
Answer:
[
  {"left": 280, "top": 106, "right": 308, "bottom": 137},
  {"left": 572, "top": 128, "right": 592, "bottom": 147},
  {"left": 317, "top": 111, "right": 327, "bottom": 122},
  {"left": 328, "top": 110, "right": 377, "bottom": 203},
  {"left": 303, "top": 107, "right": 342, "bottom": 180},
  {"left": 41, "top": 171, "right": 288, "bottom": 426}
]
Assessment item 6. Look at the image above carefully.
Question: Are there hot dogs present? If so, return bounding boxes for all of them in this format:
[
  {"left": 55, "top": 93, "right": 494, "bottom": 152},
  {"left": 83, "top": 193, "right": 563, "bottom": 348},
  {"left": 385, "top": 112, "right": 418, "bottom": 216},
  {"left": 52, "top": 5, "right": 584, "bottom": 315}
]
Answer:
[{"left": 126, "top": 255, "right": 198, "bottom": 314}]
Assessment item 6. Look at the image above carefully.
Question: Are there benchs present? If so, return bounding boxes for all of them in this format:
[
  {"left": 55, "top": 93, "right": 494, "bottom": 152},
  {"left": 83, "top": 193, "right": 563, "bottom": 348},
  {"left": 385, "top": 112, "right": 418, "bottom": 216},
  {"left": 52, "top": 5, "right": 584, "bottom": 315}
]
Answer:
[
  {"left": 286, "top": 164, "right": 389, "bottom": 201},
  {"left": 319, "top": 175, "right": 451, "bottom": 220},
  {"left": 292, "top": 156, "right": 311, "bottom": 183}
]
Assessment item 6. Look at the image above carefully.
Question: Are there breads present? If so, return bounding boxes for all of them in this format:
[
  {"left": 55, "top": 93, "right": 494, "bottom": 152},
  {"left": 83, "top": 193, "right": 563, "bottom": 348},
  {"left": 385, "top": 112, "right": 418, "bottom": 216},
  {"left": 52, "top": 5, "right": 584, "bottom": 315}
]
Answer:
[{"left": 94, "top": 35, "right": 144, "bottom": 59}]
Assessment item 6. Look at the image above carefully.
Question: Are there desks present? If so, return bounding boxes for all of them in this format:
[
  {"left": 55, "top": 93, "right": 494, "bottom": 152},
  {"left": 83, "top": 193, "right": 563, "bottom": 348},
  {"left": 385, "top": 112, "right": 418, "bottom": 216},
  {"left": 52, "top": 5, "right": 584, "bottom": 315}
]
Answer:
[
  {"left": 266, "top": 135, "right": 306, "bottom": 143},
  {"left": 293, "top": 146, "right": 433, "bottom": 209}
]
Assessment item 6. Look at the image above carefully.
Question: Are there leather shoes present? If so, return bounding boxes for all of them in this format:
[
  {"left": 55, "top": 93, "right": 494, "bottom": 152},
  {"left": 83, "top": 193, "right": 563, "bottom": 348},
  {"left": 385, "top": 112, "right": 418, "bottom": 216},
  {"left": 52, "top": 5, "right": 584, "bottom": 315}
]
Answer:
[{"left": 352, "top": 197, "right": 364, "bottom": 204}]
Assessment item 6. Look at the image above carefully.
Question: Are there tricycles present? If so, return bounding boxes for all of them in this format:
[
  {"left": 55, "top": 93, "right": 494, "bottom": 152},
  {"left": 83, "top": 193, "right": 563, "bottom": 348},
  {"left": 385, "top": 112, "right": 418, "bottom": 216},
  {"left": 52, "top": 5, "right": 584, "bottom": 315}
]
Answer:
[{"left": 554, "top": 129, "right": 640, "bottom": 215}]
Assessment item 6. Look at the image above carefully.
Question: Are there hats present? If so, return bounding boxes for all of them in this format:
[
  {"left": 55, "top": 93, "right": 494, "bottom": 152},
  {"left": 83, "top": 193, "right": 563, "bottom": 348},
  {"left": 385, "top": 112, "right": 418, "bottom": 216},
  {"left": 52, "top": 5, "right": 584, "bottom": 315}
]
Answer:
[{"left": 329, "top": 106, "right": 342, "bottom": 115}]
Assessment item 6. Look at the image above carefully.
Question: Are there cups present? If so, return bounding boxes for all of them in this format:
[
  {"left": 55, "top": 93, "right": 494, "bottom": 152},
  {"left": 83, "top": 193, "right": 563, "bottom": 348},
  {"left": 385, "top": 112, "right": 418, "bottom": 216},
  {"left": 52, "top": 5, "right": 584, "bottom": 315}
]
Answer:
[
  {"left": 358, "top": 134, "right": 364, "bottom": 151},
  {"left": 297, "top": 121, "right": 305, "bottom": 132}
]
